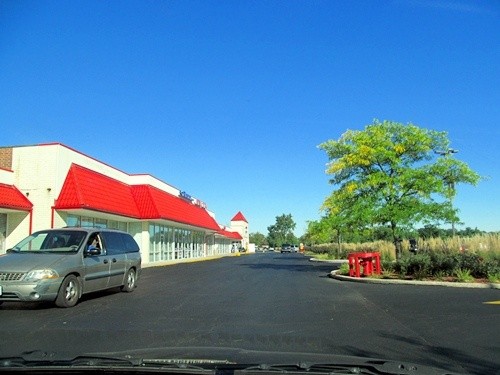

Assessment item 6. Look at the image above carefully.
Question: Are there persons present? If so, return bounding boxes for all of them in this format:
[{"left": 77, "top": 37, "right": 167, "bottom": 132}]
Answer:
[
  {"left": 87, "top": 244, "right": 95, "bottom": 250},
  {"left": 231, "top": 245, "right": 235, "bottom": 253}
]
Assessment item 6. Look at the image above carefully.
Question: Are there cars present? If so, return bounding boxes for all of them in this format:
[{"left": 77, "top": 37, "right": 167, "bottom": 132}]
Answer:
[
  {"left": 268, "top": 246, "right": 275, "bottom": 252},
  {"left": 281, "top": 243, "right": 292, "bottom": 253},
  {"left": 291, "top": 244, "right": 300, "bottom": 252}
]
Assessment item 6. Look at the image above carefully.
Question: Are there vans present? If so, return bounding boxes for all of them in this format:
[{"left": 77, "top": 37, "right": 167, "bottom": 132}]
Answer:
[{"left": 0, "top": 224, "right": 142, "bottom": 308}]
